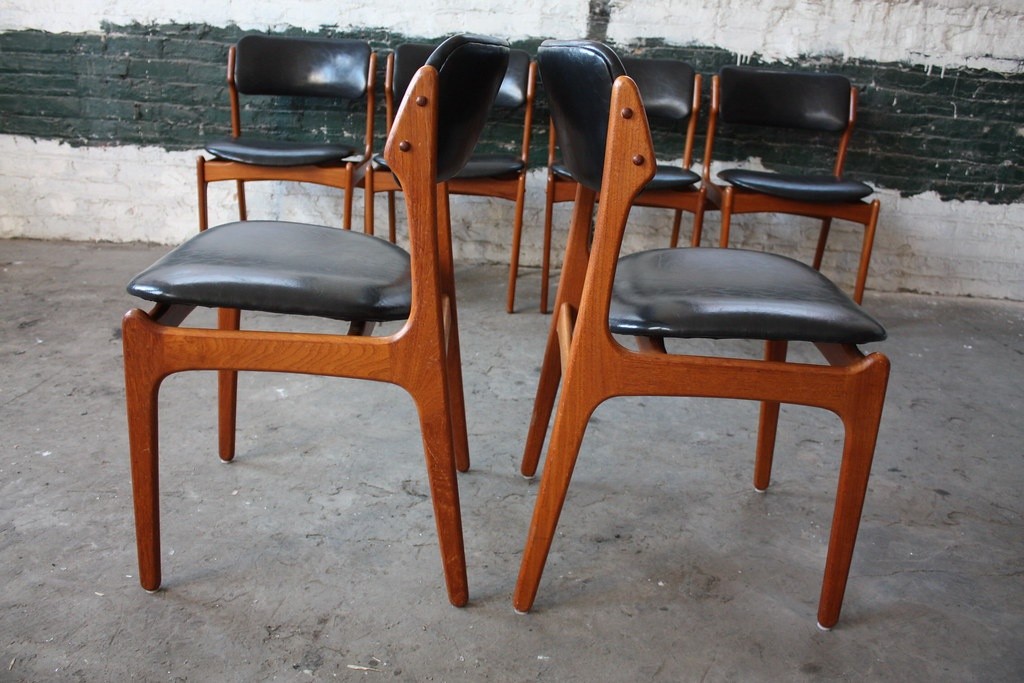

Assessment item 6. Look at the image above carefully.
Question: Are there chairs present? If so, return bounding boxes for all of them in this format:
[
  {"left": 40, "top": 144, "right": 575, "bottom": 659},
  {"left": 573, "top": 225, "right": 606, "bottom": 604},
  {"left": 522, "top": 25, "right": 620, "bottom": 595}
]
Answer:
[
  {"left": 541, "top": 59, "right": 709, "bottom": 317},
  {"left": 363, "top": 44, "right": 537, "bottom": 317},
  {"left": 514, "top": 39, "right": 890, "bottom": 630},
  {"left": 196, "top": 37, "right": 377, "bottom": 231},
  {"left": 699, "top": 65, "right": 879, "bottom": 305},
  {"left": 121, "top": 33, "right": 510, "bottom": 610}
]
